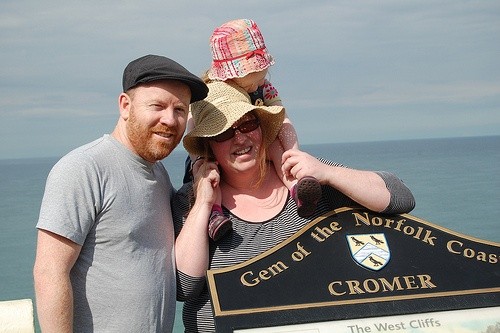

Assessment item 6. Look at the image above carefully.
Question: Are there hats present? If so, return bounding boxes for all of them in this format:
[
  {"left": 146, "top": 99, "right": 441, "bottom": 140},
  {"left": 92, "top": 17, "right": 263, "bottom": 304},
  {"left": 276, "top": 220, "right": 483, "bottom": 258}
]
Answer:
[
  {"left": 207, "top": 18, "right": 276, "bottom": 80},
  {"left": 183, "top": 79, "right": 285, "bottom": 158},
  {"left": 122, "top": 54, "right": 210, "bottom": 104}
]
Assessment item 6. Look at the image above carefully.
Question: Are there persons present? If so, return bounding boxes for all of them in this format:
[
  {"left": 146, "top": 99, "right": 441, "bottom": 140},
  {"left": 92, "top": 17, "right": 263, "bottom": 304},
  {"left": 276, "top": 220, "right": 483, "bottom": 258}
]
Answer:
[
  {"left": 176, "top": 19, "right": 320, "bottom": 243},
  {"left": 170, "top": 81, "right": 416, "bottom": 331},
  {"left": 32, "top": 55, "right": 190, "bottom": 332}
]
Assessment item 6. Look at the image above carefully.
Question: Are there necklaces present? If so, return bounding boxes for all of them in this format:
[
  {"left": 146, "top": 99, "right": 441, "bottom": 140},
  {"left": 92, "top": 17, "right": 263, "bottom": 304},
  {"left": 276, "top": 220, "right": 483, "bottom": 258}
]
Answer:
[{"left": 234, "top": 175, "right": 287, "bottom": 210}]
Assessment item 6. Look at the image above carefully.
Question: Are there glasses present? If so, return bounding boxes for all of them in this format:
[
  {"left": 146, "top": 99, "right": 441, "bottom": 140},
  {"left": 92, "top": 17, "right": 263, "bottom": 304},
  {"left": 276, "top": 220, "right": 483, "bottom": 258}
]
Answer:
[{"left": 208, "top": 118, "right": 260, "bottom": 143}]
